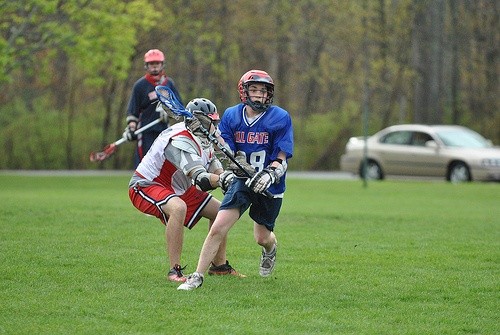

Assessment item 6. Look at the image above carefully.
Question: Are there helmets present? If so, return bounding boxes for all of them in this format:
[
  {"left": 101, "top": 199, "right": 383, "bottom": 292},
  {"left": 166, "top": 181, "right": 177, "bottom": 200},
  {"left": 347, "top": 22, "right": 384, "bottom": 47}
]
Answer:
[
  {"left": 238, "top": 69, "right": 275, "bottom": 112},
  {"left": 144, "top": 49, "right": 165, "bottom": 63},
  {"left": 184, "top": 97, "right": 220, "bottom": 136}
]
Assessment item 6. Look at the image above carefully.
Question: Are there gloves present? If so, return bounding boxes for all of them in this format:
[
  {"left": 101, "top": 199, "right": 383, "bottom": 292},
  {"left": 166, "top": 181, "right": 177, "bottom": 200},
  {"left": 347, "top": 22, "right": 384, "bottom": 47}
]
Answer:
[
  {"left": 188, "top": 115, "right": 212, "bottom": 136},
  {"left": 219, "top": 170, "right": 236, "bottom": 194},
  {"left": 245, "top": 157, "right": 288, "bottom": 194},
  {"left": 123, "top": 125, "right": 137, "bottom": 142}
]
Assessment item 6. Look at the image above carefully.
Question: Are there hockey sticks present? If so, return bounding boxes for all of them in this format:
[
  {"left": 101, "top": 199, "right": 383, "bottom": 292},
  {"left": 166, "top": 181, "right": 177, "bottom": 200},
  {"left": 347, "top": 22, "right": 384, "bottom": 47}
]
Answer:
[
  {"left": 155, "top": 85, "right": 274, "bottom": 201},
  {"left": 89, "top": 117, "right": 163, "bottom": 162}
]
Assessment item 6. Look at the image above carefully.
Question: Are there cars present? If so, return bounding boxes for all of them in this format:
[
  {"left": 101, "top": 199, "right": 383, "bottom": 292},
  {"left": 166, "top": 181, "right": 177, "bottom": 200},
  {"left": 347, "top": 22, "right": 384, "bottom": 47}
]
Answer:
[{"left": 339, "top": 124, "right": 500, "bottom": 184}]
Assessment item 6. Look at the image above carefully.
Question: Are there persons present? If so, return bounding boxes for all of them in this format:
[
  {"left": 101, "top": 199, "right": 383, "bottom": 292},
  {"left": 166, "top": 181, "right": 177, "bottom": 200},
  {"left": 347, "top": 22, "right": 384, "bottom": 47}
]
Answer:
[
  {"left": 178, "top": 69, "right": 294, "bottom": 290},
  {"left": 123, "top": 49, "right": 183, "bottom": 167},
  {"left": 127, "top": 98, "right": 247, "bottom": 283}
]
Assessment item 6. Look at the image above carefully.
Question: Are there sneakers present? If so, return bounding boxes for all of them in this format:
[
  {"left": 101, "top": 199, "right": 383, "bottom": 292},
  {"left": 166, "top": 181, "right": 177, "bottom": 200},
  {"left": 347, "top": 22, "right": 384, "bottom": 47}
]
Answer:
[
  {"left": 208, "top": 260, "right": 248, "bottom": 279},
  {"left": 177, "top": 272, "right": 204, "bottom": 292},
  {"left": 167, "top": 264, "right": 187, "bottom": 282},
  {"left": 259, "top": 245, "right": 277, "bottom": 278}
]
